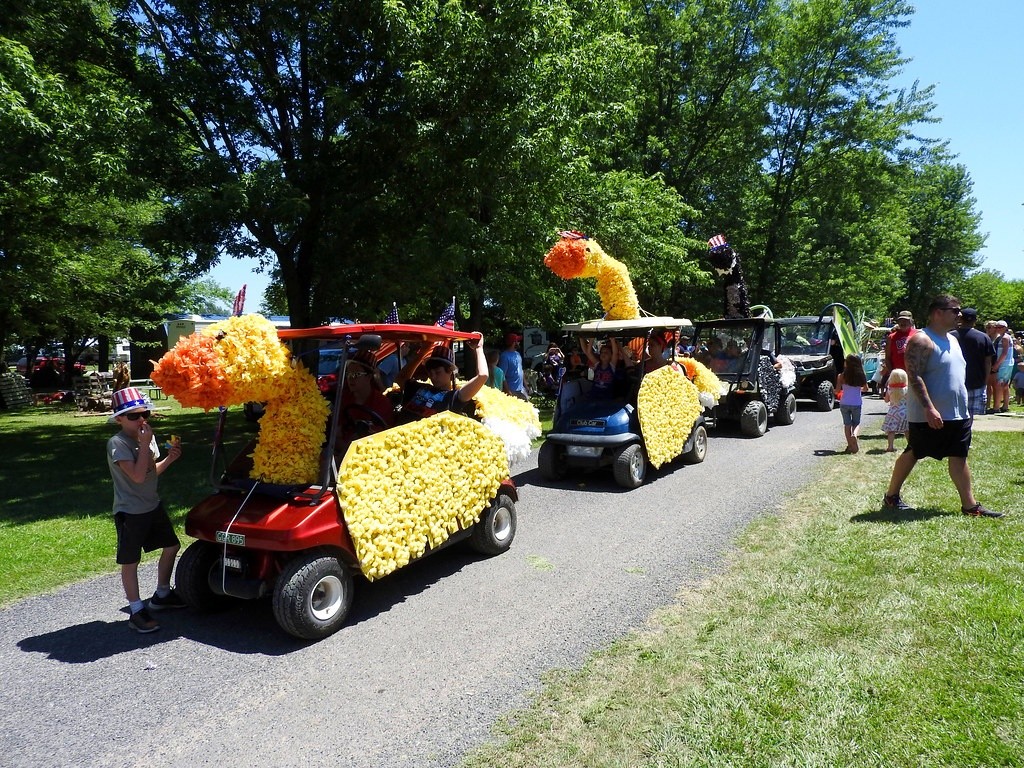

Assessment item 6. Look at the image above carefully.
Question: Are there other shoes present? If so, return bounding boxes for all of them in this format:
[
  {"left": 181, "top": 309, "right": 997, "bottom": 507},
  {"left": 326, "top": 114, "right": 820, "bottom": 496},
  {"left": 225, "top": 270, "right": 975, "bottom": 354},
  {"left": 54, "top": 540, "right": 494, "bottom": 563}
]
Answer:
[
  {"left": 845, "top": 446, "right": 854, "bottom": 453},
  {"left": 886, "top": 448, "right": 893, "bottom": 453},
  {"left": 850, "top": 434, "right": 859, "bottom": 452},
  {"left": 986, "top": 406, "right": 1010, "bottom": 414}
]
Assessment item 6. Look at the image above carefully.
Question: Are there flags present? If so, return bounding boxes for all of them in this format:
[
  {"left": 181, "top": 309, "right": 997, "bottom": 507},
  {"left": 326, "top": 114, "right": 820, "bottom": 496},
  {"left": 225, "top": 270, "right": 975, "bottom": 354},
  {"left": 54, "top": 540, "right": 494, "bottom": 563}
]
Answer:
[
  {"left": 370, "top": 307, "right": 399, "bottom": 361},
  {"left": 835, "top": 309, "right": 856, "bottom": 358},
  {"left": 435, "top": 304, "right": 455, "bottom": 360},
  {"left": 233, "top": 286, "right": 246, "bottom": 315},
  {"left": 628, "top": 326, "right": 681, "bottom": 360}
]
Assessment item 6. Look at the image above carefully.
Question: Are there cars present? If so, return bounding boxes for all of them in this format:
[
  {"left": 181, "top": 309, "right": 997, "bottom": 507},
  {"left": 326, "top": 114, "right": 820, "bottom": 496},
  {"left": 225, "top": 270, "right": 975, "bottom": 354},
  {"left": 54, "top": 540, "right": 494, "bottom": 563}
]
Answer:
[{"left": 15, "top": 336, "right": 132, "bottom": 380}]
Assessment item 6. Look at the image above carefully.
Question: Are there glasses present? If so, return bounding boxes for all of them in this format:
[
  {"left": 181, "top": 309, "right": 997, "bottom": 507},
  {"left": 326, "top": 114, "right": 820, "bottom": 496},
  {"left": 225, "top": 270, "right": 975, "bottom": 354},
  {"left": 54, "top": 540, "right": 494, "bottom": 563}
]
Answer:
[
  {"left": 994, "top": 326, "right": 1004, "bottom": 329},
  {"left": 934, "top": 307, "right": 962, "bottom": 314},
  {"left": 345, "top": 372, "right": 368, "bottom": 379},
  {"left": 118, "top": 410, "right": 151, "bottom": 421}
]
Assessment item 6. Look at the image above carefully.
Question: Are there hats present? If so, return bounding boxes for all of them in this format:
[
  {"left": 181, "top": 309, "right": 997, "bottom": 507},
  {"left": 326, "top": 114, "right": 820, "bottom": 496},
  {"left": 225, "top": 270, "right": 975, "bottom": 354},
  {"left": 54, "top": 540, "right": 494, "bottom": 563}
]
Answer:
[
  {"left": 110, "top": 387, "right": 153, "bottom": 420},
  {"left": 995, "top": 320, "right": 1008, "bottom": 328},
  {"left": 705, "top": 336, "right": 724, "bottom": 349},
  {"left": 423, "top": 346, "right": 458, "bottom": 374},
  {"left": 960, "top": 308, "right": 977, "bottom": 320},
  {"left": 345, "top": 349, "right": 380, "bottom": 375},
  {"left": 483, "top": 343, "right": 504, "bottom": 354},
  {"left": 505, "top": 333, "right": 522, "bottom": 345},
  {"left": 680, "top": 336, "right": 691, "bottom": 342},
  {"left": 724, "top": 340, "right": 741, "bottom": 352},
  {"left": 896, "top": 310, "right": 914, "bottom": 326}
]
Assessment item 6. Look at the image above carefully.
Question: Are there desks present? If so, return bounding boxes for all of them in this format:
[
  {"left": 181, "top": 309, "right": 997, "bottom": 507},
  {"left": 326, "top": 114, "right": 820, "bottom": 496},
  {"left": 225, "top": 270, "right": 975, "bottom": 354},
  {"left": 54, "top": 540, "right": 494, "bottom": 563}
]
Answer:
[{"left": 146, "top": 388, "right": 168, "bottom": 400}]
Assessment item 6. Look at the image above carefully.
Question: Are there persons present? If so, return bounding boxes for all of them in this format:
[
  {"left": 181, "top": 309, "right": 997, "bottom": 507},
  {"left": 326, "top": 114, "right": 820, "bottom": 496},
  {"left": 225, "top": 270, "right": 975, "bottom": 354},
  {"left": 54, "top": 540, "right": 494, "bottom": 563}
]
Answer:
[
  {"left": 678, "top": 337, "right": 781, "bottom": 370},
  {"left": 374, "top": 342, "right": 411, "bottom": 386},
  {"left": 781, "top": 325, "right": 838, "bottom": 358},
  {"left": 857, "top": 318, "right": 878, "bottom": 352},
  {"left": 635, "top": 331, "right": 667, "bottom": 376},
  {"left": 107, "top": 388, "right": 181, "bottom": 633},
  {"left": 543, "top": 343, "right": 581, "bottom": 386},
  {"left": 950, "top": 308, "right": 1024, "bottom": 414},
  {"left": 834, "top": 354, "right": 870, "bottom": 453},
  {"left": 485, "top": 335, "right": 529, "bottom": 401},
  {"left": 884, "top": 294, "right": 1007, "bottom": 518},
  {"left": 579, "top": 336, "right": 635, "bottom": 397},
  {"left": 872, "top": 311, "right": 920, "bottom": 451},
  {"left": 334, "top": 332, "right": 489, "bottom": 450}
]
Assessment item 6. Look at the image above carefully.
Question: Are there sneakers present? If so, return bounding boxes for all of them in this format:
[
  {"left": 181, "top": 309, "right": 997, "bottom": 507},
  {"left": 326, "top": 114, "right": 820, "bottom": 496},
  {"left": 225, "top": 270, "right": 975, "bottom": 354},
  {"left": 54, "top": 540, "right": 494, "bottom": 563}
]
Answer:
[
  {"left": 128, "top": 606, "right": 160, "bottom": 632},
  {"left": 148, "top": 584, "right": 183, "bottom": 609},
  {"left": 961, "top": 501, "right": 1005, "bottom": 519},
  {"left": 882, "top": 493, "right": 915, "bottom": 511}
]
